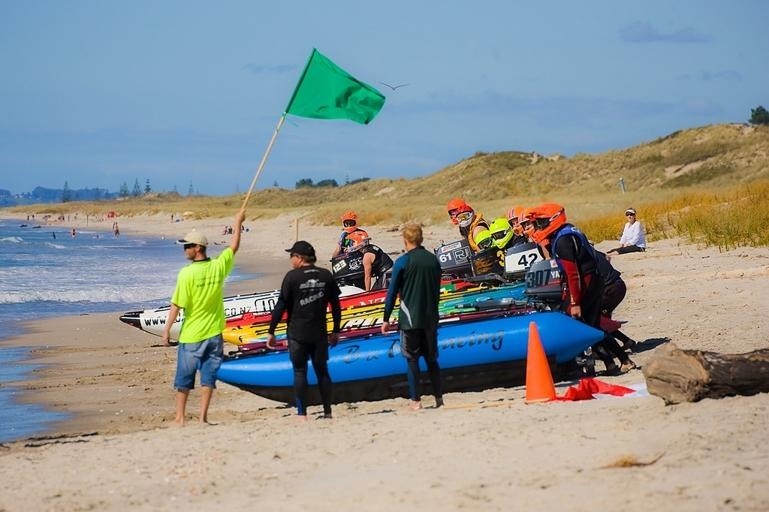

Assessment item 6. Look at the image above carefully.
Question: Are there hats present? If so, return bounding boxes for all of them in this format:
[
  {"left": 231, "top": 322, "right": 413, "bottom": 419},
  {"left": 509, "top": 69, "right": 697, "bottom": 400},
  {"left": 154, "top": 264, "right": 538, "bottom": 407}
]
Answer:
[
  {"left": 177, "top": 232, "right": 208, "bottom": 246},
  {"left": 624, "top": 209, "right": 635, "bottom": 214},
  {"left": 285, "top": 241, "right": 315, "bottom": 255}
]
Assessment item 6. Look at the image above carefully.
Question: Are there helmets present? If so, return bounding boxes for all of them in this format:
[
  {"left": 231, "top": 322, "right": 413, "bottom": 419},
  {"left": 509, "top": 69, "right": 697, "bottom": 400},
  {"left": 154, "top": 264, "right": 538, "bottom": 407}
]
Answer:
[
  {"left": 447, "top": 198, "right": 566, "bottom": 249},
  {"left": 345, "top": 231, "right": 369, "bottom": 252},
  {"left": 342, "top": 210, "right": 358, "bottom": 232}
]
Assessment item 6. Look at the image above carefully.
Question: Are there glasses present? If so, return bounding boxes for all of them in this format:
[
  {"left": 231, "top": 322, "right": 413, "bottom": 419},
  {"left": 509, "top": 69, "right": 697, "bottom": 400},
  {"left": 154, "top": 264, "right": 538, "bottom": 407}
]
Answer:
[{"left": 626, "top": 214, "right": 635, "bottom": 216}]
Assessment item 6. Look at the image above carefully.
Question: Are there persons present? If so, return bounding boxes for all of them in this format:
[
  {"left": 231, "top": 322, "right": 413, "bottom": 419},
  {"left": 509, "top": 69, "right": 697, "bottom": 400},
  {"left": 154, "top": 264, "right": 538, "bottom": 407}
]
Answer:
[
  {"left": 382, "top": 223, "right": 446, "bottom": 414},
  {"left": 162, "top": 208, "right": 246, "bottom": 426},
  {"left": 605, "top": 208, "right": 646, "bottom": 256},
  {"left": 344, "top": 232, "right": 397, "bottom": 292},
  {"left": 447, "top": 197, "right": 639, "bottom": 379},
  {"left": 266, "top": 240, "right": 343, "bottom": 420},
  {"left": 26, "top": 212, "right": 120, "bottom": 239},
  {"left": 331, "top": 211, "right": 371, "bottom": 291},
  {"left": 224, "top": 222, "right": 245, "bottom": 234}
]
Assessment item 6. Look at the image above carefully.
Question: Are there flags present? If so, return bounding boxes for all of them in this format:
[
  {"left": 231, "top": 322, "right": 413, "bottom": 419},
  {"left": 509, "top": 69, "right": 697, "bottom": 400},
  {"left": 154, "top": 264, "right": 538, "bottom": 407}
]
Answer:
[{"left": 281, "top": 47, "right": 386, "bottom": 127}]
